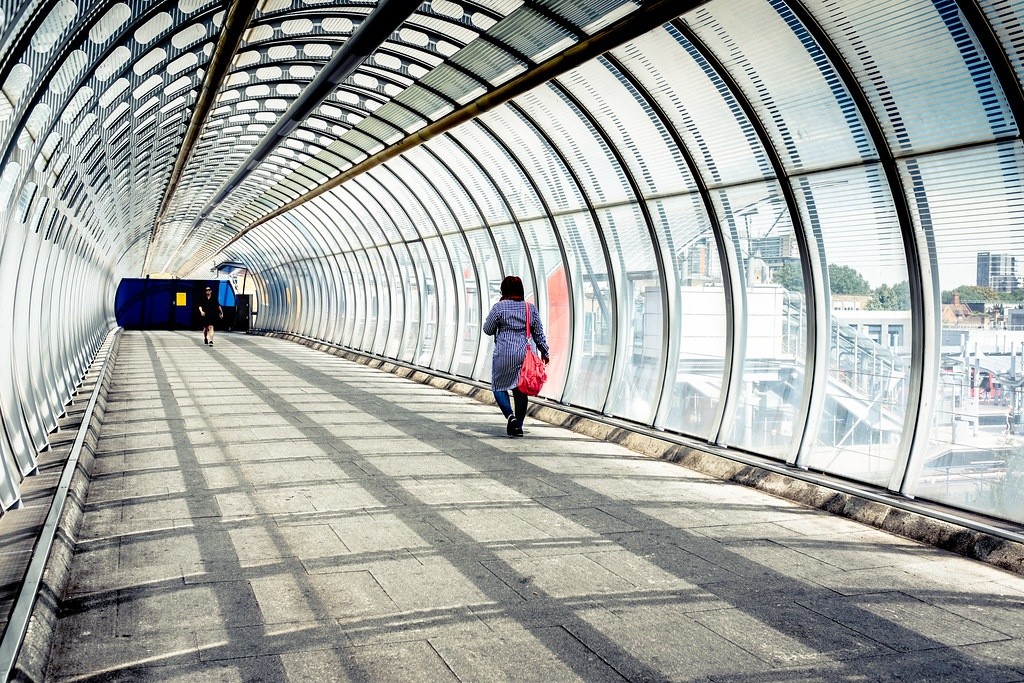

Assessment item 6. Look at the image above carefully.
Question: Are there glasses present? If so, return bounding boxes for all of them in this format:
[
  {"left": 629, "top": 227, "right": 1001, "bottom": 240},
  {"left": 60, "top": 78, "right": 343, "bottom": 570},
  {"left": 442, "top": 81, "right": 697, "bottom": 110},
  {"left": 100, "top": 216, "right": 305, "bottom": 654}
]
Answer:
[{"left": 206, "top": 289, "right": 211, "bottom": 292}]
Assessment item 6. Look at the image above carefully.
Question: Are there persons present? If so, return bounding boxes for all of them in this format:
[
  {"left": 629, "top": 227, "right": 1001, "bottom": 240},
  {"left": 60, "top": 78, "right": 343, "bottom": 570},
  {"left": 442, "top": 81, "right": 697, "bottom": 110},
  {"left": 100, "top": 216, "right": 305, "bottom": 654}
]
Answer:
[
  {"left": 483, "top": 276, "right": 550, "bottom": 437},
  {"left": 199, "top": 287, "right": 223, "bottom": 346}
]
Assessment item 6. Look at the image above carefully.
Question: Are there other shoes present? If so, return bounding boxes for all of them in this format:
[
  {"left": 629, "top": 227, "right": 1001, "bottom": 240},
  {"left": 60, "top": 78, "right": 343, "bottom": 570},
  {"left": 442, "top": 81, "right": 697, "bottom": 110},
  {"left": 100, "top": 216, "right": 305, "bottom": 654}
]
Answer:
[
  {"left": 204, "top": 338, "right": 208, "bottom": 345},
  {"left": 209, "top": 340, "right": 214, "bottom": 347}
]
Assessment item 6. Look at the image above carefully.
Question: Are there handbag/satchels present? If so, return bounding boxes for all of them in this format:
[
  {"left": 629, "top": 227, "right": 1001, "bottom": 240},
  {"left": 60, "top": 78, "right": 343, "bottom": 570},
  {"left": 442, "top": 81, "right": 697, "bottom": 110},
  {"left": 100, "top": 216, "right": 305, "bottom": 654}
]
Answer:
[{"left": 518, "top": 344, "right": 547, "bottom": 396}]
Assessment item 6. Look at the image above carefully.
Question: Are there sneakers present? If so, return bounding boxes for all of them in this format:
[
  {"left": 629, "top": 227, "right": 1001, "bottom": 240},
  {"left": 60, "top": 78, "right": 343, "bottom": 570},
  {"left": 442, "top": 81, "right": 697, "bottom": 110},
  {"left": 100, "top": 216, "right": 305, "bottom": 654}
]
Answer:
[
  {"left": 507, "top": 414, "right": 517, "bottom": 436},
  {"left": 512, "top": 428, "right": 523, "bottom": 437}
]
